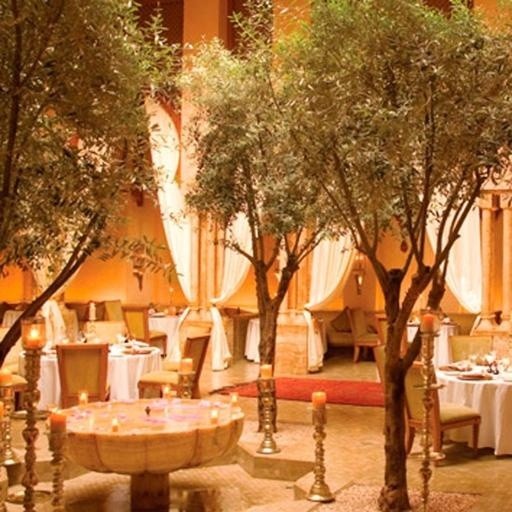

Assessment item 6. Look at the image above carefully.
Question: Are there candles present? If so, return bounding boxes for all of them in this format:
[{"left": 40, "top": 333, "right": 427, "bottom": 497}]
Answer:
[
  {"left": 311, "top": 391, "right": 327, "bottom": 410},
  {"left": 260, "top": 364, "right": 273, "bottom": 378}
]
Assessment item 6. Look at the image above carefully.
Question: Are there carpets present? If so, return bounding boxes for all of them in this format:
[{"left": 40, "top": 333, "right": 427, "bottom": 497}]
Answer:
[{"left": 223, "top": 377, "right": 386, "bottom": 408}]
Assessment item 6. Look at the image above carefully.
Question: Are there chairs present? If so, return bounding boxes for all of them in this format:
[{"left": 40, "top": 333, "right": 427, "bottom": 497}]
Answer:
[
  {"left": 3, "top": 303, "right": 257, "bottom": 417},
  {"left": 371, "top": 308, "right": 511, "bottom": 455},
  {"left": 248, "top": 302, "right": 384, "bottom": 373}
]
